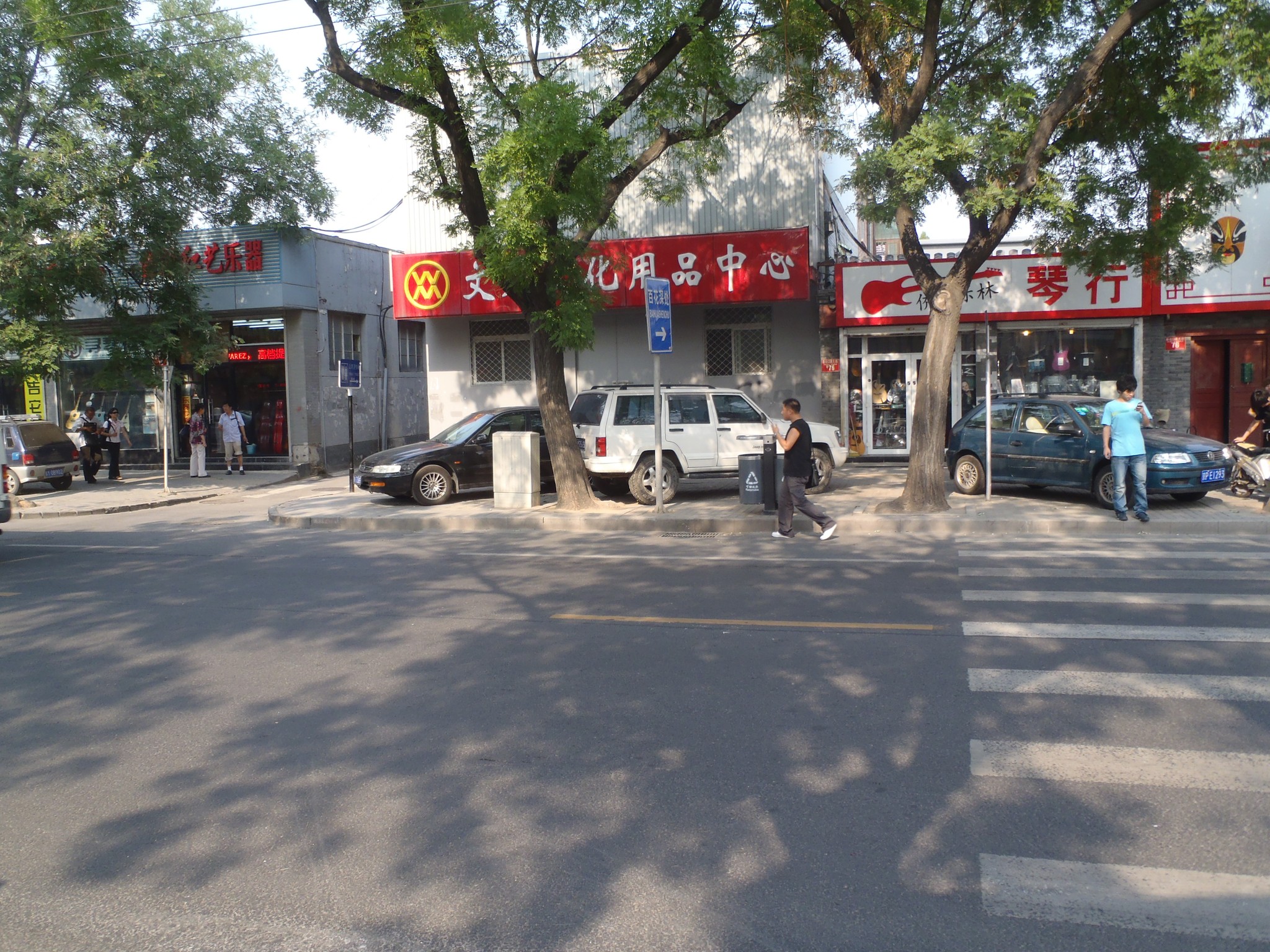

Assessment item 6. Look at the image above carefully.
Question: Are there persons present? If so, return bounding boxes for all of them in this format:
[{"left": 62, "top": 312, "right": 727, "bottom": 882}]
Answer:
[
  {"left": 1234, "top": 376, "right": 1270, "bottom": 447},
  {"left": 189, "top": 403, "right": 211, "bottom": 478},
  {"left": 771, "top": 398, "right": 838, "bottom": 540},
  {"left": 218, "top": 402, "right": 248, "bottom": 475},
  {"left": 100, "top": 407, "right": 132, "bottom": 481},
  {"left": 962, "top": 381, "right": 976, "bottom": 410},
  {"left": 1101, "top": 372, "right": 1153, "bottom": 522},
  {"left": 71, "top": 407, "right": 105, "bottom": 484}
]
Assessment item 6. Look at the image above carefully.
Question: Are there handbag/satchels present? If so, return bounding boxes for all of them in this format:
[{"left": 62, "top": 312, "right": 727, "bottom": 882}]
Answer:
[
  {"left": 82, "top": 422, "right": 99, "bottom": 447},
  {"left": 100, "top": 437, "right": 107, "bottom": 449},
  {"left": 241, "top": 434, "right": 250, "bottom": 452},
  {"left": 805, "top": 464, "right": 819, "bottom": 489}
]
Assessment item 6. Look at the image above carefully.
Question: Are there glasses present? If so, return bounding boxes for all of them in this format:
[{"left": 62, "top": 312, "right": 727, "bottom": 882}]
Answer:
[
  {"left": 222, "top": 407, "right": 225, "bottom": 409},
  {"left": 113, "top": 412, "right": 118, "bottom": 414}
]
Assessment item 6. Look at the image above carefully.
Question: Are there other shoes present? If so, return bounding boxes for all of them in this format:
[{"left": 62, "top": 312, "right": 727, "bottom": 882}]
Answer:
[
  {"left": 109, "top": 476, "right": 123, "bottom": 479},
  {"left": 1135, "top": 512, "right": 1150, "bottom": 521},
  {"left": 226, "top": 470, "right": 232, "bottom": 475},
  {"left": 820, "top": 523, "right": 837, "bottom": 540},
  {"left": 240, "top": 470, "right": 245, "bottom": 475},
  {"left": 191, "top": 475, "right": 198, "bottom": 478},
  {"left": 772, "top": 531, "right": 790, "bottom": 538},
  {"left": 199, "top": 475, "right": 210, "bottom": 477},
  {"left": 88, "top": 476, "right": 96, "bottom": 484},
  {"left": 1115, "top": 511, "right": 1128, "bottom": 520}
]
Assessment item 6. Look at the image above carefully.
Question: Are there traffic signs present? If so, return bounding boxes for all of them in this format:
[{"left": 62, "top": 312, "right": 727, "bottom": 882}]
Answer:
[{"left": 643, "top": 275, "right": 673, "bottom": 354}]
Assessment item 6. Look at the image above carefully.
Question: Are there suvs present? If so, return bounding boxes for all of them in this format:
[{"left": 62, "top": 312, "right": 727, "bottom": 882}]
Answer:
[
  {"left": 570, "top": 383, "right": 850, "bottom": 506},
  {"left": 945, "top": 390, "right": 1237, "bottom": 511}
]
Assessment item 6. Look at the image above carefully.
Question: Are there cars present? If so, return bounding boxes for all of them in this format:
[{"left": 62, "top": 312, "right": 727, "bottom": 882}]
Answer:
[
  {"left": 0, "top": 414, "right": 81, "bottom": 496},
  {"left": 352, "top": 405, "right": 558, "bottom": 506}
]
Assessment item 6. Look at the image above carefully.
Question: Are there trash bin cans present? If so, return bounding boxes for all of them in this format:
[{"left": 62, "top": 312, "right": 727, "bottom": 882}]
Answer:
[
  {"left": 774, "top": 454, "right": 785, "bottom": 504},
  {"left": 737, "top": 453, "right": 765, "bottom": 505}
]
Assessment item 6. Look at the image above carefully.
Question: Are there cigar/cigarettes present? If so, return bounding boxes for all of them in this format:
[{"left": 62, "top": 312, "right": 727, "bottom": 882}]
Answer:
[{"left": 247, "top": 442, "right": 249, "bottom": 444}]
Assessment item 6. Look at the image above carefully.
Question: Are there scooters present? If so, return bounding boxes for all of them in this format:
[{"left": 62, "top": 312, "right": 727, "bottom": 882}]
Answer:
[{"left": 1227, "top": 429, "right": 1270, "bottom": 499}]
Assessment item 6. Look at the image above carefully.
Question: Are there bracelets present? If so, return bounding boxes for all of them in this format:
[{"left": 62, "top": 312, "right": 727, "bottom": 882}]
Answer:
[
  {"left": 774, "top": 431, "right": 779, "bottom": 439},
  {"left": 1246, "top": 427, "right": 1251, "bottom": 434}
]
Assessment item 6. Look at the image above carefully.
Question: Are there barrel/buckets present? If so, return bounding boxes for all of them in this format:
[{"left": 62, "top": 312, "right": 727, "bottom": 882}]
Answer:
[{"left": 246, "top": 445, "right": 256, "bottom": 454}]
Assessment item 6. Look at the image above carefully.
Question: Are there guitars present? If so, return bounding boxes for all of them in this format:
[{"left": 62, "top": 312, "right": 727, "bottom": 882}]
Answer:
[
  {"left": 871, "top": 361, "right": 887, "bottom": 405},
  {"left": 851, "top": 358, "right": 862, "bottom": 377},
  {"left": 873, "top": 409, "right": 887, "bottom": 448},
  {"left": 887, "top": 366, "right": 906, "bottom": 404},
  {"left": 848, "top": 411, "right": 866, "bottom": 456},
  {"left": 111, "top": 392, "right": 119, "bottom": 408},
  {"left": 89, "top": 394, "right": 107, "bottom": 435},
  {"left": 119, "top": 394, "right": 135, "bottom": 434},
  {"left": 84, "top": 393, "right": 96, "bottom": 414},
  {"left": 1078, "top": 330, "right": 1097, "bottom": 373},
  {"left": 1025, "top": 332, "right": 1046, "bottom": 373},
  {"left": 1051, "top": 330, "right": 1071, "bottom": 371},
  {"left": 65, "top": 391, "right": 84, "bottom": 429}
]
